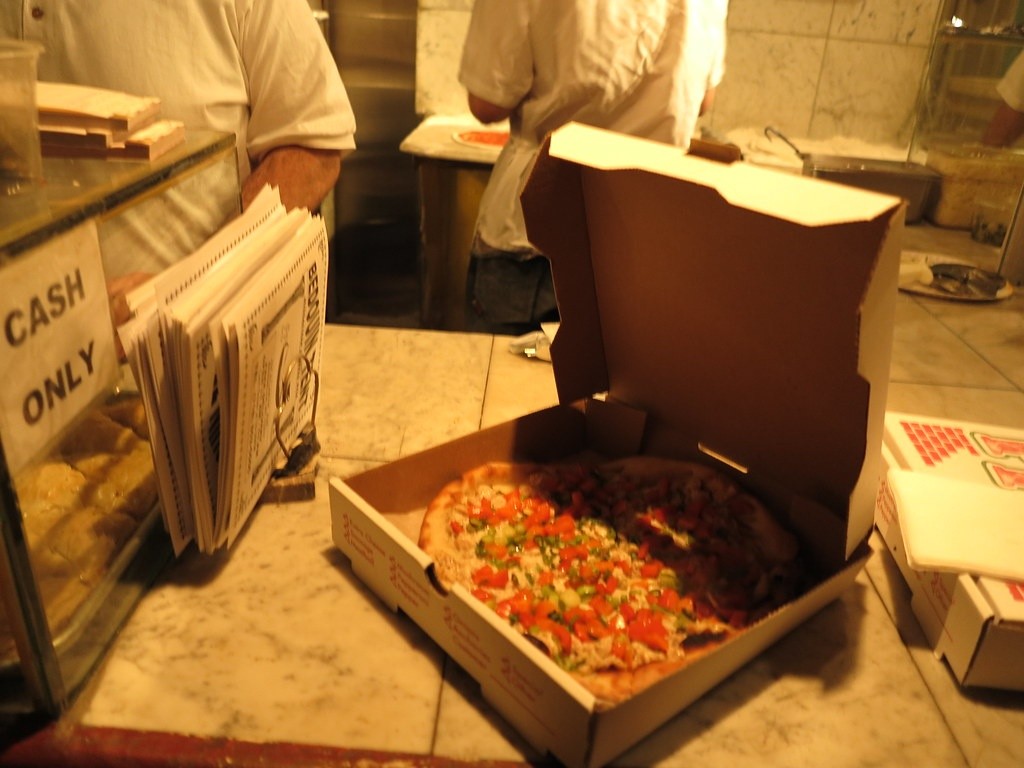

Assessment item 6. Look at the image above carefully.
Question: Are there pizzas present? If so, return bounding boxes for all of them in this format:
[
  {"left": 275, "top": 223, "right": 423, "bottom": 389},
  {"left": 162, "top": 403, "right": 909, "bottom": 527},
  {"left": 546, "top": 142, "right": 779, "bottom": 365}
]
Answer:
[
  {"left": 419, "top": 453, "right": 797, "bottom": 706},
  {"left": 453, "top": 128, "right": 513, "bottom": 151}
]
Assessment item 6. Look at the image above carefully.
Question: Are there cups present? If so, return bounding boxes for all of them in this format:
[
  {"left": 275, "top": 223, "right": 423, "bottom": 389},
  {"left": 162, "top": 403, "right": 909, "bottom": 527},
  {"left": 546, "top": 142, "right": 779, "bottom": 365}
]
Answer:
[{"left": 1, "top": 42, "right": 44, "bottom": 195}]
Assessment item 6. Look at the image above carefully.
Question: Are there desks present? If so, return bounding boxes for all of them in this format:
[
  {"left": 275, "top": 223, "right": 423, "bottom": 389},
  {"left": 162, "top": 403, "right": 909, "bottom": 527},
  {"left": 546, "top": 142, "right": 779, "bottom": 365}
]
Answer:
[{"left": 399, "top": 114, "right": 806, "bottom": 337}]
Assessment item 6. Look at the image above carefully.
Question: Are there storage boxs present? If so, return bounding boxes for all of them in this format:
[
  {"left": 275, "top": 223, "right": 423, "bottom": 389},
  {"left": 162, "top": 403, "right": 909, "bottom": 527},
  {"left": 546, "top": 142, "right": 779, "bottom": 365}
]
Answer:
[
  {"left": 866, "top": 468, "right": 1024, "bottom": 697},
  {"left": 321, "top": 119, "right": 903, "bottom": 763}
]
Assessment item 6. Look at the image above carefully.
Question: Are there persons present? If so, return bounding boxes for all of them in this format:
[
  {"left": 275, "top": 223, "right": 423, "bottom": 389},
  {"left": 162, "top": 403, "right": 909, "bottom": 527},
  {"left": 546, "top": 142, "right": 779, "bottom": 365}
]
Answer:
[
  {"left": 457, "top": 1, "right": 730, "bottom": 338},
  {"left": 1, "top": 1, "right": 358, "bottom": 369}
]
